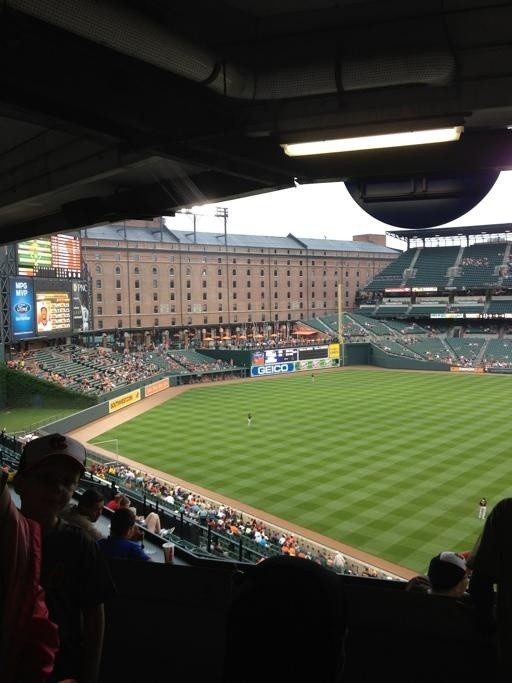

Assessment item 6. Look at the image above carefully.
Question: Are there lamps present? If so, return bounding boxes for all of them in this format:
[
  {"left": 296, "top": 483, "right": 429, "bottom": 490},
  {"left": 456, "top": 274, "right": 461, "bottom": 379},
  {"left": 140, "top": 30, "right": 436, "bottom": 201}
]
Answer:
[{"left": 278, "top": 109, "right": 469, "bottom": 157}]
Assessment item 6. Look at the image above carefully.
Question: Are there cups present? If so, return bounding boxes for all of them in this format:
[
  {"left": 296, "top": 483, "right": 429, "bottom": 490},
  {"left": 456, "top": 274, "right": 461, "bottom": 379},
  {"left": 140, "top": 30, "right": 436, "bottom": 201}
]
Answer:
[{"left": 162, "top": 542, "right": 175, "bottom": 562}]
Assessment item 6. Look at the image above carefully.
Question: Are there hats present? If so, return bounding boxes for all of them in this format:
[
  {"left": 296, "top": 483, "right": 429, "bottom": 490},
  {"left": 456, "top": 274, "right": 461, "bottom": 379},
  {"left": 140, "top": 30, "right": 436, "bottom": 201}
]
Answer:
[
  {"left": 21, "top": 433, "right": 88, "bottom": 473},
  {"left": 428, "top": 551, "right": 471, "bottom": 591}
]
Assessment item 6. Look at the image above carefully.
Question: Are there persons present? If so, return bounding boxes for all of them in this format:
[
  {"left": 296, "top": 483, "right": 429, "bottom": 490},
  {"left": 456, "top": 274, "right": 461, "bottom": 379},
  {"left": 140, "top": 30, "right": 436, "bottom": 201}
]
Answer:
[{"left": 248, "top": 413, "right": 253, "bottom": 426}]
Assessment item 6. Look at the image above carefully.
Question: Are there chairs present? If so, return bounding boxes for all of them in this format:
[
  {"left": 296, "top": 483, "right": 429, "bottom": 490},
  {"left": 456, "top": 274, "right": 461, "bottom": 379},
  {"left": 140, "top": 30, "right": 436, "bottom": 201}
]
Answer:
[
  {"left": 300, "top": 243, "right": 512, "bottom": 365},
  {"left": 152, "top": 479, "right": 241, "bottom": 575},
  {"left": 2, "top": 445, "right": 156, "bottom": 574},
  {"left": 8, "top": 341, "right": 246, "bottom": 395},
  {"left": 237, "top": 515, "right": 356, "bottom": 575}
]
